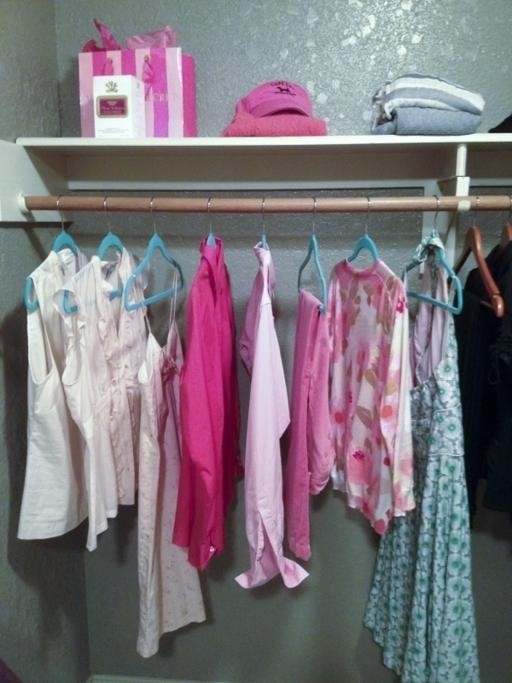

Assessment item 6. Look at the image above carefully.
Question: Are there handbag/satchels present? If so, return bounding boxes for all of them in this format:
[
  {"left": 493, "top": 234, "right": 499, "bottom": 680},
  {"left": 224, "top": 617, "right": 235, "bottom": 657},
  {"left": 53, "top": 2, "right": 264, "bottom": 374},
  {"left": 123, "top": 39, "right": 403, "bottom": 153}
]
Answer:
[{"left": 79, "top": 19, "right": 196, "bottom": 137}]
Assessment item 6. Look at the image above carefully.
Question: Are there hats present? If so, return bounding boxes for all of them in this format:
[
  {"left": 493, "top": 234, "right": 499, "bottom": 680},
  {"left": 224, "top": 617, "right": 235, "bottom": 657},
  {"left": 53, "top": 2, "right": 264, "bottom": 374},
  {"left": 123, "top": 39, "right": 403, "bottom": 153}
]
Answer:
[{"left": 241, "top": 81, "right": 312, "bottom": 118}]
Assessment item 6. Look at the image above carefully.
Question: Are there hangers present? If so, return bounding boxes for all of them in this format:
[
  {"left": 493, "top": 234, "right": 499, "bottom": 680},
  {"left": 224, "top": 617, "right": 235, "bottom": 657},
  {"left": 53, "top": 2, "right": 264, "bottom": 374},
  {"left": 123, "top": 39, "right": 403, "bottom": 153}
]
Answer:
[{"left": 22, "top": 189, "right": 504, "bottom": 319}]
